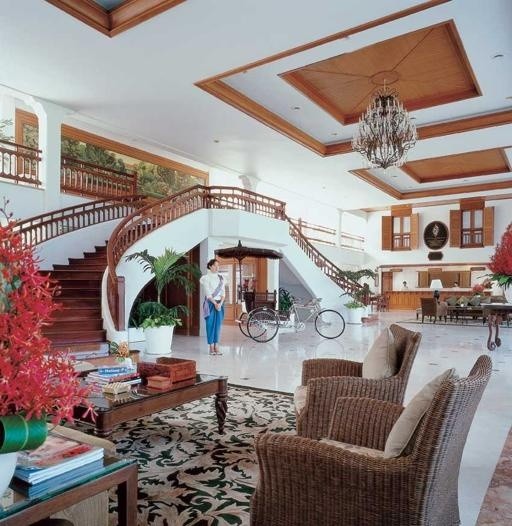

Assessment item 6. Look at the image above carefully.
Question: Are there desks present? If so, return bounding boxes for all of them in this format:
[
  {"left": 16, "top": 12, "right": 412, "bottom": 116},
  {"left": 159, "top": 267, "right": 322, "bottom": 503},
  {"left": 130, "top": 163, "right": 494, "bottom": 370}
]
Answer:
[
  {"left": 0, "top": 418, "right": 144, "bottom": 525},
  {"left": 66, "top": 366, "right": 232, "bottom": 445},
  {"left": 483, "top": 300, "right": 512, "bottom": 351},
  {"left": 443, "top": 302, "right": 487, "bottom": 324}
]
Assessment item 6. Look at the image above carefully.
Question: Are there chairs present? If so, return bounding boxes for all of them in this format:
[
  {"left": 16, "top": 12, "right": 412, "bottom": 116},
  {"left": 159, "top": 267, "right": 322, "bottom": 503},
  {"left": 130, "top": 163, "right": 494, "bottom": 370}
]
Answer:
[
  {"left": 292, "top": 325, "right": 426, "bottom": 435},
  {"left": 242, "top": 285, "right": 277, "bottom": 321},
  {"left": 420, "top": 293, "right": 448, "bottom": 324},
  {"left": 253, "top": 349, "right": 492, "bottom": 525},
  {"left": 364, "top": 290, "right": 390, "bottom": 314}
]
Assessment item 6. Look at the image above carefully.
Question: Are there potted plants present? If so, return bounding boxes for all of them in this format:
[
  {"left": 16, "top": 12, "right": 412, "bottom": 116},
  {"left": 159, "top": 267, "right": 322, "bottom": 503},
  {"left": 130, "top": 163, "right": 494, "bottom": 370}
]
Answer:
[
  {"left": 337, "top": 265, "right": 378, "bottom": 325},
  {"left": 278, "top": 285, "right": 295, "bottom": 324},
  {"left": 124, "top": 247, "right": 203, "bottom": 355}
]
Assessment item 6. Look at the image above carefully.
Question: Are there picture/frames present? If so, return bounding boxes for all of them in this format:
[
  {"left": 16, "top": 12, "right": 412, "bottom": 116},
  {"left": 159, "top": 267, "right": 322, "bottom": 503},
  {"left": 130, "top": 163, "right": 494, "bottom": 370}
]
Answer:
[{"left": 14, "top": 104, "right": 214, "bottom": 208}]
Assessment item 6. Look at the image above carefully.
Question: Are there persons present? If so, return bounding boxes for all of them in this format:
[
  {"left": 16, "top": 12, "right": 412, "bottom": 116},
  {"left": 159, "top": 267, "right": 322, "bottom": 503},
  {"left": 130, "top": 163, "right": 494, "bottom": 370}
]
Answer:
[
  {"left": 199, "top": 259, "right": 225, "bottom": 355},
  {"left": 403, "top": 280, "right": 411, "bottom": 291},
  {"left": 452, "top": 281, "right": 461, "bottom": 288}
]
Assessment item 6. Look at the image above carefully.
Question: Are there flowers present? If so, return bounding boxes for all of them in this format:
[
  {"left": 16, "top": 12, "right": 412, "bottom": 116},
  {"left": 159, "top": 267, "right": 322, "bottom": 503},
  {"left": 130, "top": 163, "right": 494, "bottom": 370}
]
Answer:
[
  {"left": 481, "top": 220, "right": 512, "bottom": 292},
  {"left": 0, "top": 199, "right": 100, "bottom": 451}
]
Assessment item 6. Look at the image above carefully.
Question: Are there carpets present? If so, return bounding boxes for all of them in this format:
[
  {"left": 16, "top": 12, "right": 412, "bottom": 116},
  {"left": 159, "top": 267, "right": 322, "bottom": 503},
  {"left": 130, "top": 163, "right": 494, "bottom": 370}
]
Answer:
[{"left": 56, "top": 376, "right": 325, "bottom": 526}]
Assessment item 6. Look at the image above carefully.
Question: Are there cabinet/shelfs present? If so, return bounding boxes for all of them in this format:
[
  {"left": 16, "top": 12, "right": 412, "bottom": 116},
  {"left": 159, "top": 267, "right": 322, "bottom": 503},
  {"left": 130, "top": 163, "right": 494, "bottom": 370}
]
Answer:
[
  {"left": 386, "top": 290, "right": 494, "bottom": 313},
  {"left": 215, "top": 253, "right": 236, "bottom": 326},
  {"left": 234, "top": 255, "right": 268, "bottom": 327}
]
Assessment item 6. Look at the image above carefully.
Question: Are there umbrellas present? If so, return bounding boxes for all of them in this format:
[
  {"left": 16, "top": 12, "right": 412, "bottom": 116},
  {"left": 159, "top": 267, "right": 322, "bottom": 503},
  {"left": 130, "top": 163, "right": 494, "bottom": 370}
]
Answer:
[{"left": 214, "top": 239, "right": 284, "bottom": 301}]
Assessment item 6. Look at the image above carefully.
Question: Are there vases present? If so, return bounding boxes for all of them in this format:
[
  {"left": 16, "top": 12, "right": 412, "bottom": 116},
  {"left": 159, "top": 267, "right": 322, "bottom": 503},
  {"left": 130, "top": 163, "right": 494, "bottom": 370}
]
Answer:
[{"left": 0, "top": 446, "right": 19, "bottom": 502}]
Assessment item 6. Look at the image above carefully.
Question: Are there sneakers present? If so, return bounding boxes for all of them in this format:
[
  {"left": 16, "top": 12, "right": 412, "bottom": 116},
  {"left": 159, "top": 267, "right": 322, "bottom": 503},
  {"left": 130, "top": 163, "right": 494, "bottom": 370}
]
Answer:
[{"left": 209, "top": 352, "right": 222, "bottom": 355}]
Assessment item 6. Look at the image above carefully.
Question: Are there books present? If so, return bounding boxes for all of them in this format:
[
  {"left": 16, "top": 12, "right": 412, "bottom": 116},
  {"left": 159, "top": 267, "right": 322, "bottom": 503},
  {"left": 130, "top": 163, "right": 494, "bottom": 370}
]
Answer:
[
  {"left": 84, "top": 361, "right": 142, "bottom": 392},
  {"left": 16, "top": 443, "right": 105, "bottom": 485},
  {"left": 9, "top": 458, "right": 105, "bottom": 499}
]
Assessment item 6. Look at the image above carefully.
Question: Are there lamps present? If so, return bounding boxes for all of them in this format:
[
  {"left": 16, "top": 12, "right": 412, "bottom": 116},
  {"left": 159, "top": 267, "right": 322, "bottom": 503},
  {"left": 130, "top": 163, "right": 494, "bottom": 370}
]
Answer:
[
  {"left": 430, "top": 278, "right": 442, "bottom": 299},
  {"left": 354, "top": 78, "right": 422, "bottom": 171}
]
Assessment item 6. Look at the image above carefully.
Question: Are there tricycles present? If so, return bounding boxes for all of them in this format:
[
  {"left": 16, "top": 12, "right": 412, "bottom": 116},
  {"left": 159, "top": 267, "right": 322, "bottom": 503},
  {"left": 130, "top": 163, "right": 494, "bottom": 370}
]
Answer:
[{"left": 234, "top": 288, "right": 346, "bottom": 343}]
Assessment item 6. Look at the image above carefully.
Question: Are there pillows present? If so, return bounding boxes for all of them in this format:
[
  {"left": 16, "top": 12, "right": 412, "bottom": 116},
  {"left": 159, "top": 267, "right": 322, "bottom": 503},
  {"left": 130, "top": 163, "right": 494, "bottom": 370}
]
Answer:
[
  {"left": 467, "top": 294, "right": 480, "bottom": 308},
  {"left": 458, "top": 298, "right": 469, "bottom": 308},
  {"left": 381, "top": 366, "right": 457, "bottom": 460},
  {"left": 480, "top": 295, "right": 491, "bottom": 302},
  {"left": 446, "top": 295, "right": 458, "bottom": 305},
  {"left": 363, "top": 328, "right": 400, "bottom": 381}
]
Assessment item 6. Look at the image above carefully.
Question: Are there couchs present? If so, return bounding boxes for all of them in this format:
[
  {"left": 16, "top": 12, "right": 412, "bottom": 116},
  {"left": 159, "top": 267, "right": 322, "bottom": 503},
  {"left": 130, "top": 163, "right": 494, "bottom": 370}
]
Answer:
[{"left": 418, "top": 291, "right": 507, "bottom": 325}]
